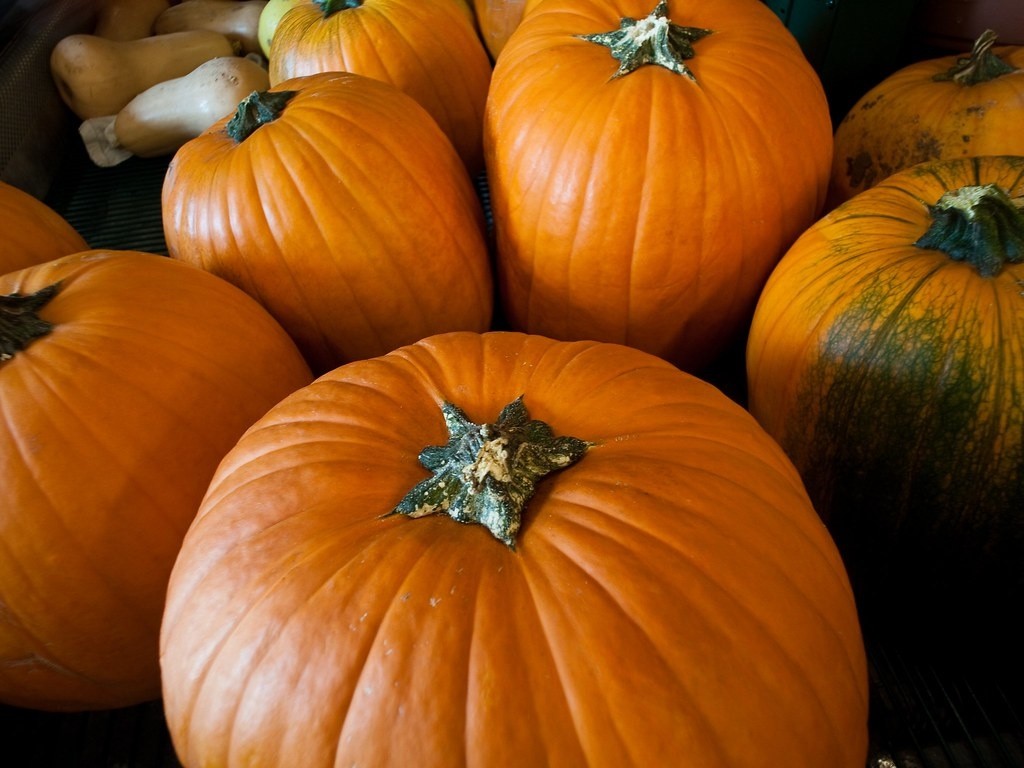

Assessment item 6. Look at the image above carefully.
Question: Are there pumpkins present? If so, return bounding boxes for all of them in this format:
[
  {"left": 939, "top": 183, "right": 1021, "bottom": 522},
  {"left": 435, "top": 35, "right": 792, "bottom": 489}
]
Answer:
[{"left": 0, "top": 0, "right": 1024, "bottom": 768}]
